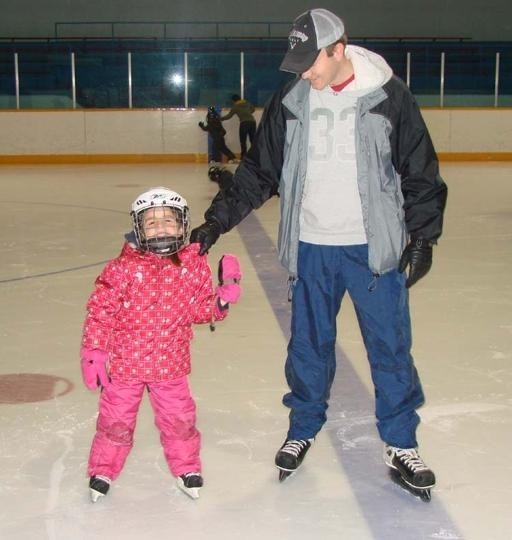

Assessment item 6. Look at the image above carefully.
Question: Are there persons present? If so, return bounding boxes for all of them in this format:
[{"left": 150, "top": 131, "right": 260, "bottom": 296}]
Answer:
[
  {"left": 189, "top": 6, "right": 450, "bottom": 490},
  {"left": 198, "top": 93, "right": 258, "bottom": 164},
  {"left": 80, "top": 185, "right": 243, "bottom": 495}
]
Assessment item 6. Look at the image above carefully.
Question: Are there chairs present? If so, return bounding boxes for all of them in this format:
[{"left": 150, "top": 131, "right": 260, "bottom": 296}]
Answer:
[
  {"left": 178, "top": 471, "right": 204, "bottom": 489},
  {"left": 272, "top": 434, "right": 316, "bottom": 473},
  {"left": 383, "top": 440, "right": 437, "bottom": 491},
  {"left": 88, "top": 473, "right": 113, "bottom": 496}
]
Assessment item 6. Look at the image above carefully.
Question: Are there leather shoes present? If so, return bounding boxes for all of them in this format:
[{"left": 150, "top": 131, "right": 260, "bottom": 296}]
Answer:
[
  {"left": 398, "top": 236, "right": 434, "bottom": 290},
  {"left": 215, "top": 254, "right": 243, "bottom": 304},
  {"left": 80, "top": 346, "right": 111, "bottom": 391},
  {"left": 191, "top": 219, "right": 221, "bottom": 255}
]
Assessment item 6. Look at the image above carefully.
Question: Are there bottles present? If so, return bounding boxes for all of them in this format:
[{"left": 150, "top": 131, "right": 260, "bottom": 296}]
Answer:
[{"left": 278, "top": 7, "right": 346, "bottom": 74}]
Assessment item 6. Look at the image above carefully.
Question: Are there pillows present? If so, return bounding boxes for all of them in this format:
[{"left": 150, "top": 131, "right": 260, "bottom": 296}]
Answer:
[{"left": 130, "top": 185, "right": 191, "bottom": 213}]
